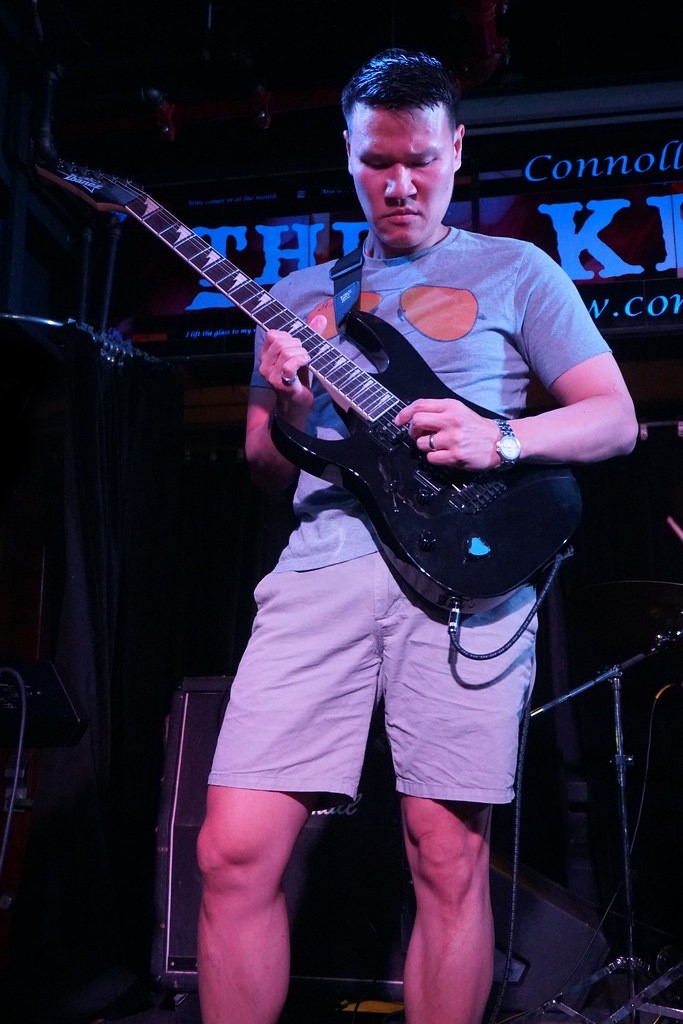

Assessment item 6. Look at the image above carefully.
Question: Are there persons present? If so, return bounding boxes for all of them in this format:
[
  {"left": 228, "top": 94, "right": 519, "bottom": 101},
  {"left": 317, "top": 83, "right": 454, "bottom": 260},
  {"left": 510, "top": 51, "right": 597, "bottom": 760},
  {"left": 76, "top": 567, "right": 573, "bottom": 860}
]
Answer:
[{"left": 197, "top": 50, "right": 641, "bottom": 1024}]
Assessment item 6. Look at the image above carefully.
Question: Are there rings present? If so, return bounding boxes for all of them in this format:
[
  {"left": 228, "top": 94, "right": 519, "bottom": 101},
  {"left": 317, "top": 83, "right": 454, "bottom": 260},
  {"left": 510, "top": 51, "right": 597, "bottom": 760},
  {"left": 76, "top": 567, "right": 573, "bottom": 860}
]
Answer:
[
  {"left": 429, "top": 435, "right": 435, "bottom": 449},
  {"left": 281, "top": 374, "right": 297, "bottom": 386}
]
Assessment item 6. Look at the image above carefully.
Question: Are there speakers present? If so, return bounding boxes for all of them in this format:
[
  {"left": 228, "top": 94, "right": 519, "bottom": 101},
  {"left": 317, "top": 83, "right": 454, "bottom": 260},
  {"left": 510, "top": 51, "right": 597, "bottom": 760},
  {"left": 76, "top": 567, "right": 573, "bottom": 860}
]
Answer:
[
  {"left": 153, "top": 670, "right": 422, "bottom": 1001},
  {"left": 480, "top": 834, "right": 620, "bottom": 1023}
]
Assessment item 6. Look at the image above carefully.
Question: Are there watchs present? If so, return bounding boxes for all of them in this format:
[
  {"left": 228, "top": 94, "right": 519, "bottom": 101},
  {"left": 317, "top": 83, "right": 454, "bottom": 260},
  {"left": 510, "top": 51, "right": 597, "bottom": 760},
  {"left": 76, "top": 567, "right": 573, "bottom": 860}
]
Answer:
[{"left": 493, "top": 417, "right": 522, "bottom": 466}]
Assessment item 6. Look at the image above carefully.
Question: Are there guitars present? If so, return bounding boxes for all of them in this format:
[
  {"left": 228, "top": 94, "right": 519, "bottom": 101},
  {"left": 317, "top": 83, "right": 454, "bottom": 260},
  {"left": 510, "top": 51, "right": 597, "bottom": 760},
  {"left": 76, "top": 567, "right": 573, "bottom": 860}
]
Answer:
[{"left": 31, "top": 156, "right": 587, "bottom": 618}]
